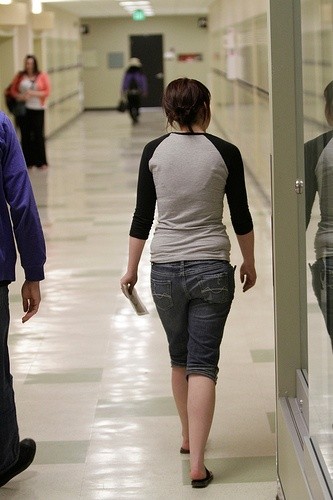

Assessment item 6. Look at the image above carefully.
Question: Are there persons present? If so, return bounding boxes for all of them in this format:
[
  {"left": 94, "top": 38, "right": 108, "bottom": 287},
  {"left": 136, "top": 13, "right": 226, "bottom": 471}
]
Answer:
[
  {"left": 0, "top": 112, "right": 46, "bottom": 489},
  {"left": 120, "top": 58, "right": 147, "bottom": 126},
  {"left": 10, "top": 56, "right": 50, "bottom": 169},
  {"left": 304, "top": 80, "right": 333, "bottom": 351},
  {"left": 120, "top": 78, "right": 257, "bottom": 488}
]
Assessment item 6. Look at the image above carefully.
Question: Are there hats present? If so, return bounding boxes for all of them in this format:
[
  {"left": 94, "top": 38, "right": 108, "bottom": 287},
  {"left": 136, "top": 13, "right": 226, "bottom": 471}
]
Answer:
[{"left": 127, "top": 58, "right": 141, "bottom": 67}]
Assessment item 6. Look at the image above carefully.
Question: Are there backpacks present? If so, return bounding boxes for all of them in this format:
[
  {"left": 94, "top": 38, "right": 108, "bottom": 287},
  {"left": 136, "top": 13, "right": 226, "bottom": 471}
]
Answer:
[
  {"left": 6, "top": 85, "right": 16, "bottom": 112},
  {"left": 128, "top": 79, "right": 139, "bottom": 95}
]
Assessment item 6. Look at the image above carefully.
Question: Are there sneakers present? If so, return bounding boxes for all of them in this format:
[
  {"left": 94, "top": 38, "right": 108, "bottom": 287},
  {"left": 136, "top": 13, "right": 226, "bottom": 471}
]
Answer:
[{"left": 0, "top": 439, "right": 36, "bottom": 488}]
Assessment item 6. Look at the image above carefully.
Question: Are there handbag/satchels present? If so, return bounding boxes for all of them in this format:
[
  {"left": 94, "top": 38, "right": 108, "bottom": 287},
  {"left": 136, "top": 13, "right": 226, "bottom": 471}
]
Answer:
[
  {"left": 12, "top": 102, "right": 26, "bottom": 116},
  {"left": 120, "top": 100, "right": 126, "bottom": 112}
]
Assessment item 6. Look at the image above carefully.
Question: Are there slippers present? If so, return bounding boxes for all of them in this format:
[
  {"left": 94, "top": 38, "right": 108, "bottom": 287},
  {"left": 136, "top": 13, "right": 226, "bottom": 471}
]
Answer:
[
  {"left": 190, "top": 465, "right": 213, "bottom": 488},
  {"left": 180, "top": 447, "right": 189, "bottom": 453}
]
